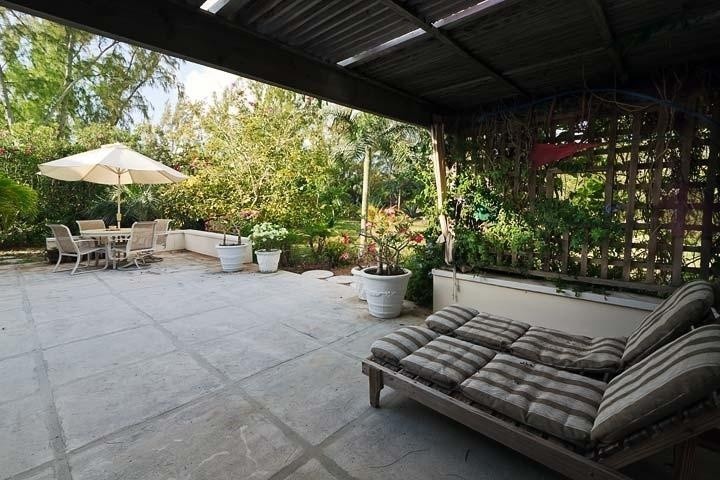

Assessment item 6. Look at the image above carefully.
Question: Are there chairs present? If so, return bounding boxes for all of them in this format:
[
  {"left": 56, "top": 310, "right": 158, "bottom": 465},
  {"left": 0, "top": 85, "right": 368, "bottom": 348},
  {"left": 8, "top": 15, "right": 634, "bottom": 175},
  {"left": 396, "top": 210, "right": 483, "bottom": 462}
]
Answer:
[
  {"left": 44, "top": 218, "right": 174, "bottom": 274},
  {"left": 362, "top": 324, "right": 720, "bottom": 479},
  {"left": 425, "top": 279, "right": 718, "bottom": 382}
]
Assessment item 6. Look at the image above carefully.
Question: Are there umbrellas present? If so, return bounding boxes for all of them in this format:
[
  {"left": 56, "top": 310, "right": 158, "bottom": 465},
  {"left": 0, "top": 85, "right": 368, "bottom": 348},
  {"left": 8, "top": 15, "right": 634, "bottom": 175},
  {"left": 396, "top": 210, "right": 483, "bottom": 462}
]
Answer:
[{"left": 36, "top": 141, "right": 189, "bottom": 263}]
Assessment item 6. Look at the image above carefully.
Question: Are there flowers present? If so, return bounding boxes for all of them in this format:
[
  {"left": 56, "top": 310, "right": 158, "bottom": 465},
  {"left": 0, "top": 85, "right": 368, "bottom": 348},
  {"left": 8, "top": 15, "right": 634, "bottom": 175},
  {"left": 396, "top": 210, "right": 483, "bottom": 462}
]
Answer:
[
  {"left": 202, "top": 206, "right": 289, "bottom": 251},
  {"left": 339, "top": 205, "right": 425, "bottom": 276}
]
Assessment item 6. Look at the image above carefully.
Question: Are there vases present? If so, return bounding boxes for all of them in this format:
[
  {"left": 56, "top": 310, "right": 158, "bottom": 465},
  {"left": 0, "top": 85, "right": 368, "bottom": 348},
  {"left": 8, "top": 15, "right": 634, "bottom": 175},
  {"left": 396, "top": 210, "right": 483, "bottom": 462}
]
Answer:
[
  {"left": 215, "top": 242, "right": 282, "bottom": 274},
  {"left": 352, "top": 264, "right": 412, "bottom": 318}
]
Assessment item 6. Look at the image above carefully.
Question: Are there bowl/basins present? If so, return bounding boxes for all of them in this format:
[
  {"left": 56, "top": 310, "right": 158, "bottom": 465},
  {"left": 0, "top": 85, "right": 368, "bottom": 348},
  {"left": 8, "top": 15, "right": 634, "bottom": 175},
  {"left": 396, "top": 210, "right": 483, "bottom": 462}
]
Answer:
[{"left": 109, "top": 226, "right": 117, "bottom": 231}]
